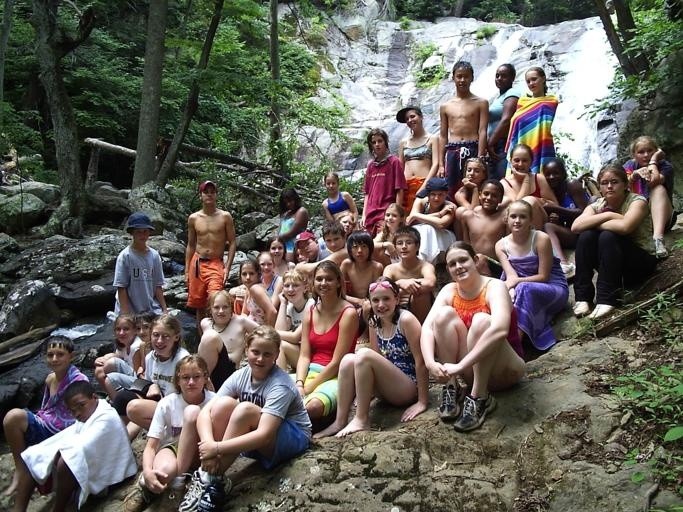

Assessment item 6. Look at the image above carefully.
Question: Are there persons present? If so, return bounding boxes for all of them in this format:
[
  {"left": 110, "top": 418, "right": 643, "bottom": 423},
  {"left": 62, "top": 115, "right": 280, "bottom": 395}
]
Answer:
[
  {"left": 2, "top": 336, "right": 89, "bottom": 496},
  {"left": 622, "top": 136, "right": 677, "bottom": 260},
  {"left": 14, "top": 381, "right": 139, "bottom": 512},
  {"left": 571, "top": 164, "right": 657, "bottom": 320}
]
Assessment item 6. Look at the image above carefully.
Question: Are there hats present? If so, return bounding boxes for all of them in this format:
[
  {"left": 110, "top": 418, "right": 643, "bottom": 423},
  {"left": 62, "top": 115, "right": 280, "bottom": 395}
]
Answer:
[
  {"left": 395, "top": 105, "right": 422, "bottom": 124},
  {"left": 415, "top": 177, "right": 449, "bottom": 198},
  {"left": 199, "top": 180, "right": 218, "bottom": 192},
  {"left": 126, "top": 212, "right": 156, "bottom": 232},
  {"left": 294, "top": 232, "right": 316, "bottom": 247}
]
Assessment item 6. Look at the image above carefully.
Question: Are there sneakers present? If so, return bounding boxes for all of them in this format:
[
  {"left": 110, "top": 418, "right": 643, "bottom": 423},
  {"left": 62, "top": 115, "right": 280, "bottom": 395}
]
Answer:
[
  {"left": 572, "top": 301, "right": 590, "bottom": 316},
  {"left": 179, "top": 469, "right": 232, "bottom": 511},
  {"left": 588, "top": 304, "right": 615, "bottom": 320},
  {"left": 439, "top": 383, "right": 461, "bottom": 419},
  {"left": 124, "top": 473, "right": 154, "bottom": 510},
  {"left": 653, "top": 236, "right": 668, "bottom": 259},
  {"left": 453, "top": 393, "right": 497, "bottom": 432}
]
